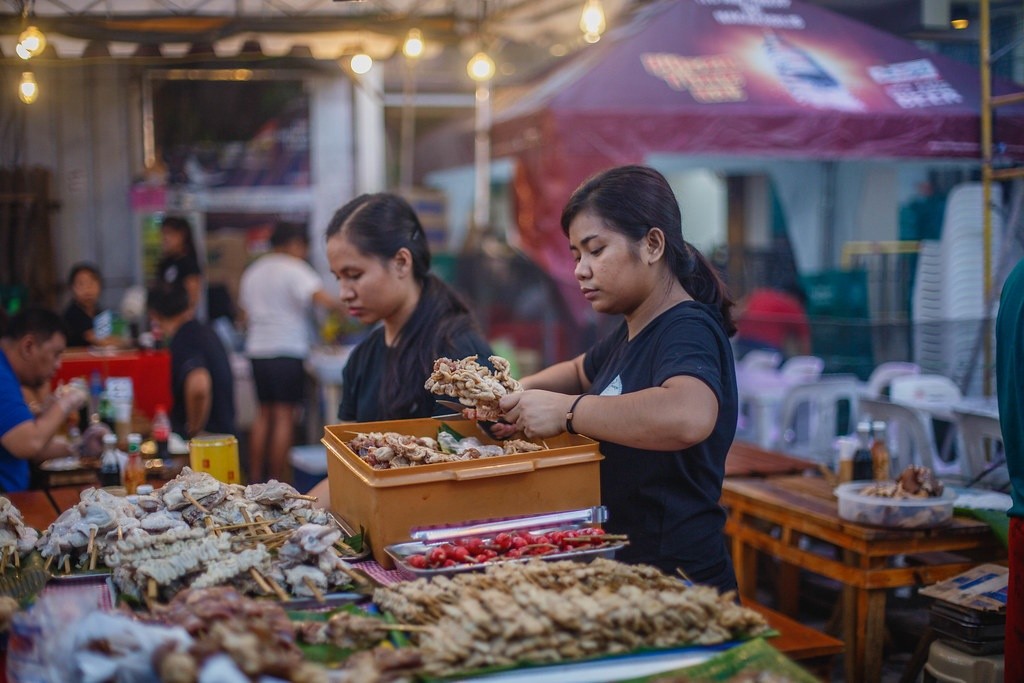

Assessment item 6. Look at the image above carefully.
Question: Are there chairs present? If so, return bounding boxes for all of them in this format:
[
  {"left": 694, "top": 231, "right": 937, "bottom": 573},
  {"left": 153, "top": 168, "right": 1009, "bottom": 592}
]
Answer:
[{"left": 731, "top": 343, "right": 1013, "bottom": 496}]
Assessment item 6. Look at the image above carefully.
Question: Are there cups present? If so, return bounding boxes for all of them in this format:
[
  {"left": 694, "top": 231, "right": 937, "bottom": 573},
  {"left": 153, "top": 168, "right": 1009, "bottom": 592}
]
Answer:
[{"left": 840, "top": 444, "right": 853, "bottom": 481}]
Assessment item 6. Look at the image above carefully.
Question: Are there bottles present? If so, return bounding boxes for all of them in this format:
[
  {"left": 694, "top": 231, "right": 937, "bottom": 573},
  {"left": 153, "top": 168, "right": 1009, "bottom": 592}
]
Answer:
[
  {"left": 125, "top": 434, "right": 145, "bottom": 495},
  {"left": 65, "top": 373, "right": 116, "bottom": 435},
  {"left": 152, "top": 405, "right": 173, "bottom": 462},
  {"left": 152, "top": 320, "right": 164, "bottom": 351},
  {"left": 853, "top": 423, "right": 873, "bottom": 482},
  {"left": 871, "top": 421, "right": 891, "bottom": 481},
  {"left": 99, "top": 436, "right": 121, "bottom": 486}
]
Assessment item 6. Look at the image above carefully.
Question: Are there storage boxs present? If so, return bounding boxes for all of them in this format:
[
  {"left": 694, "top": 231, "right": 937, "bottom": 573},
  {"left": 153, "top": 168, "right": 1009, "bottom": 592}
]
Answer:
[
  {"left": 321, "top": 413, "right": 606, "bottom": 570},
  {"left": 51, "top": 344, "right": 174, "bottom": 422}
]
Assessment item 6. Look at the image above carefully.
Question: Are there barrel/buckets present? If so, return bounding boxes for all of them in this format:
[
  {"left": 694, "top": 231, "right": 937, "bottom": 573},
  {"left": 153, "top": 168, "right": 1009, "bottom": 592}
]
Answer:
[{"left": 190, "top": 435, "right": 240, "bottom": 487}]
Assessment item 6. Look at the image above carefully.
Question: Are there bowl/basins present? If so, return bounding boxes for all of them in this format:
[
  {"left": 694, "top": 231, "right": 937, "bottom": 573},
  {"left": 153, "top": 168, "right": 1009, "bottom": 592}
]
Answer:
[{"left": 834, "top": 483, "right": 956, "bottom": 529}]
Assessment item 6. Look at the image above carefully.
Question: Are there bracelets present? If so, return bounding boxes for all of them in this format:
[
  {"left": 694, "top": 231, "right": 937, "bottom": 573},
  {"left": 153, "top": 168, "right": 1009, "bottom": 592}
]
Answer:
[{"left": 565, "top": 393, "right": 593, "bottom": 434}]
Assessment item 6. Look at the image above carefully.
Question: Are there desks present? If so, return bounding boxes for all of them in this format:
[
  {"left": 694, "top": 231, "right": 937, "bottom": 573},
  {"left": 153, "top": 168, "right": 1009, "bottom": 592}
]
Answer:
[{"left": 717, "top": 468, "right": 995, "bottom": 683}]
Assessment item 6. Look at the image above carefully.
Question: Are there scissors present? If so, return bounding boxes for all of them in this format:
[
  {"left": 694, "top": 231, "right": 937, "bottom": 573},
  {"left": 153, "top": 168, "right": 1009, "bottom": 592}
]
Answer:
[{"left": 431, "top": 400, "right": 514, "bottom": 441}]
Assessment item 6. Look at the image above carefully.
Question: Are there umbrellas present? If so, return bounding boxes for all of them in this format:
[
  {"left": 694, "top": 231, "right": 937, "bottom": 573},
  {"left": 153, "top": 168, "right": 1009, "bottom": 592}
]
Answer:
[{"left": 419, "top": 0, "right": 1023, "bottom": 356}]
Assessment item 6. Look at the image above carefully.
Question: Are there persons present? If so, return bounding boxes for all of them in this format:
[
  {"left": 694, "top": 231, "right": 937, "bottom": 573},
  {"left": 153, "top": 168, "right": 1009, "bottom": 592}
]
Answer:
[
  {"left": 142, "top": 283, "right": 235, "bottom": 481},
  {"left": 734, "top": 278, "right": 811, "bottom": 367},
  {"left": 50, "top": 261, "right": 138, "bottom": 350},
  {"left": 476, "top": 164, "right": 741, "bottom": 608},
  {"left": 0, "top": 300, "right": 87, "bottom": 493},
  {"left": 995, "top": 255, "right": 1024, "bottom": 683},
  {"left": 236, "top": 222, "right": 347, "bottom": 486},
  {"left": 304, "top": 194, "right": 500, "bottom": 509},
  {"left": 148, "top": 217, "right": 201, "bottom": 350}
]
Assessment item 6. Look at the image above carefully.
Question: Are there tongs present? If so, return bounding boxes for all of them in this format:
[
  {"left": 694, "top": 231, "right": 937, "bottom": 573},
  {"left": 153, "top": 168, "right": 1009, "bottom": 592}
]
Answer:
[{"left": 411, "top": 506, "right": 608, "bottom": 544}]
